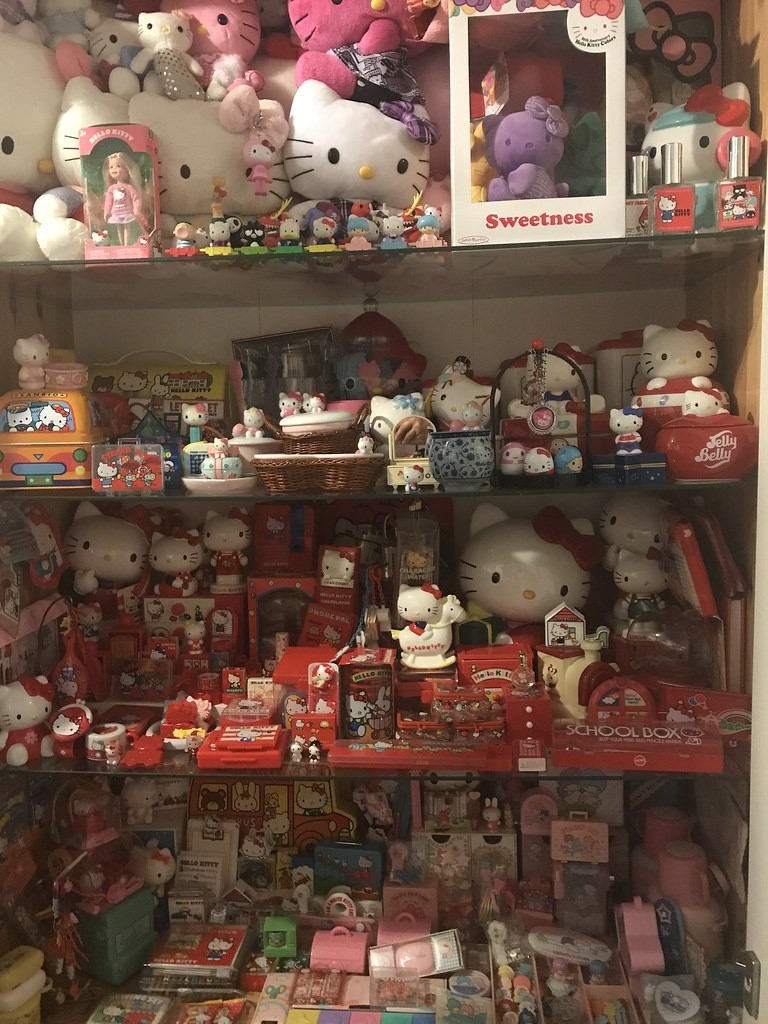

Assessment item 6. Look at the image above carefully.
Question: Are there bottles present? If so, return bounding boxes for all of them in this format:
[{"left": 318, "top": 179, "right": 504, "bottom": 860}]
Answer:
[
  {"left": 630, "top": 806, "right": 690, "bottom": 904},
  {"left": 647, "top": 842, "right": 730, "bottom": 964}
]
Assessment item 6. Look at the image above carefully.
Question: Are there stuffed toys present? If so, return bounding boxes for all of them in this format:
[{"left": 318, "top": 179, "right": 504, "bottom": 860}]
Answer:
[{"left": 0, "top": 0, "right": 570, "bottom": 277}]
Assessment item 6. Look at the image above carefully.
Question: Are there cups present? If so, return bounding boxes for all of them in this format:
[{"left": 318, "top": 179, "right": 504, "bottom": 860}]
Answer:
[{"left": 428, "top": 429, "right": 495, "bottom": 490}]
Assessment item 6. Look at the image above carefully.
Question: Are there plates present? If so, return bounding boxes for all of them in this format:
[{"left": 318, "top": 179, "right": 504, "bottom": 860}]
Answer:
[{"left": 181, "top": 474, "right": 258, "bottom": 494}]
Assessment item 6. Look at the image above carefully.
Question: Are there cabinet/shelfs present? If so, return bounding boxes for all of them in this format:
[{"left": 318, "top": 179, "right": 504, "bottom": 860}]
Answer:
[{"left": 0, "top": 227, "right": 763, "bottom": 779}]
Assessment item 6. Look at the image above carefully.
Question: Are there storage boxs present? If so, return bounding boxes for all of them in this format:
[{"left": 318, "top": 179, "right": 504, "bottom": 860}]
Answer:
[{"left": 0, "top": 0, "right": 760, "bottom": 1024}]
